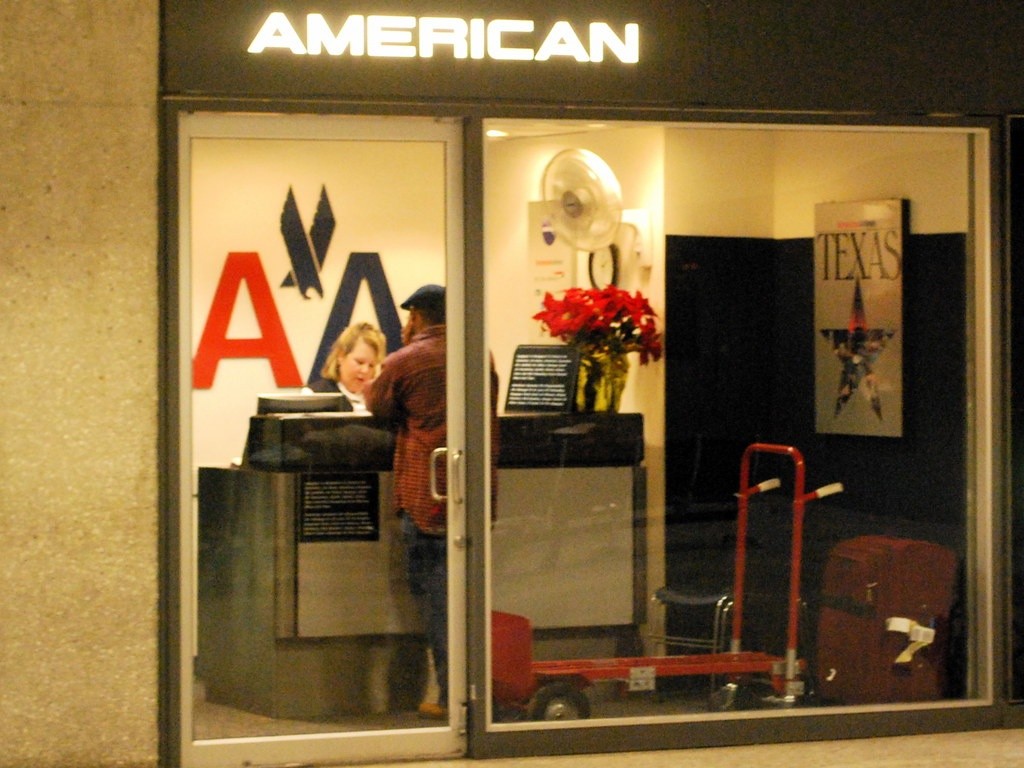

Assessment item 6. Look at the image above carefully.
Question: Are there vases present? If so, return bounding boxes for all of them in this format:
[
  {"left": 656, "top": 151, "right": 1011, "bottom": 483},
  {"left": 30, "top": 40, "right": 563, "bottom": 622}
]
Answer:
[{"left": 573, "top": 342, "right": 628, "bottom": 416}]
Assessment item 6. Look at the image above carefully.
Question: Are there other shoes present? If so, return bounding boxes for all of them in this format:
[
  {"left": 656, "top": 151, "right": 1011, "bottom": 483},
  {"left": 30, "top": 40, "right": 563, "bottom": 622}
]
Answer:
[{"left": 418, "top": 699, "right": 450, "bottom": 721}]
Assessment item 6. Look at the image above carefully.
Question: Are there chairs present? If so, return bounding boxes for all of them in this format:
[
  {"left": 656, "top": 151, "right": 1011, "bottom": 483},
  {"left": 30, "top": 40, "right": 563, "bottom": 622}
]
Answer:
[{"left": 655, "top": 491, "right": 825, "bottom": 713}]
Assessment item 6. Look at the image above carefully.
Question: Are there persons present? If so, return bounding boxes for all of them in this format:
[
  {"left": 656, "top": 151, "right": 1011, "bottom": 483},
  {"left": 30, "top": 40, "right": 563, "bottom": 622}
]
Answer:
[
  {"left": 306, "top": 323, "right": 388, "bottom": 414},
  {"left": 366, "top": 284, "right": 500, "bottom": 720}
]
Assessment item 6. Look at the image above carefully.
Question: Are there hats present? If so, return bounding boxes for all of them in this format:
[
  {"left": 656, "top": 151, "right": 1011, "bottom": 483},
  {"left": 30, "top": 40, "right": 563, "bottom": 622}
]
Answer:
[{"left": 400, "top": 284, "right": 445, "bottom": 312}]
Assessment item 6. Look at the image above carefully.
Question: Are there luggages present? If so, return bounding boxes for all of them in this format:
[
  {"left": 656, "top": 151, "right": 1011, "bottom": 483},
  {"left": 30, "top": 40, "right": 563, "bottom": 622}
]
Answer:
[{"left": 811, "top": 536, "right": 959, "bottom": 701}]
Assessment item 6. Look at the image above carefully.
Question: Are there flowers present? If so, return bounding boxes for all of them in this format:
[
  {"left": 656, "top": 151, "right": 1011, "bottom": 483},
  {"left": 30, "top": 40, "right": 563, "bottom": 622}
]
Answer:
[{"left": 541, "top": 286, "right": 666, "bottom": 365}]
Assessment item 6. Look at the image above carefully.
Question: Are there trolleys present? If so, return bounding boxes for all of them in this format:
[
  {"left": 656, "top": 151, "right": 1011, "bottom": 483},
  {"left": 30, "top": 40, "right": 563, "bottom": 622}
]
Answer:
[{"left": 490, "top": 442, "right": 843, "bottom": 723}]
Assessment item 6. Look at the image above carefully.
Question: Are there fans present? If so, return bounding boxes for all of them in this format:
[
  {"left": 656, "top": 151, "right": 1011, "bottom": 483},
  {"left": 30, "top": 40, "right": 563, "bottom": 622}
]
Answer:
[{"left": 542, "top": 145, "right": 652, "bottom": 270}]
